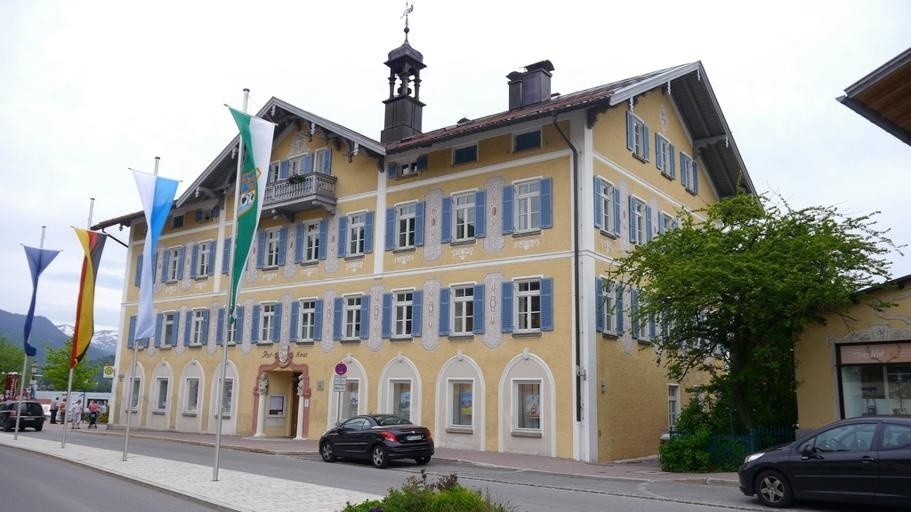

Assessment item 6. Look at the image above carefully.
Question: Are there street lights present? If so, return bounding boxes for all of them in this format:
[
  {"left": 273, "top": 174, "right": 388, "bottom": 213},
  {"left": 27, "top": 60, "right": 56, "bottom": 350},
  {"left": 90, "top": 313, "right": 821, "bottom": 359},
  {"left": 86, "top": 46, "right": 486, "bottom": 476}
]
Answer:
[{"left": 30, "top": 359, "right": 39, "bottom": 399}]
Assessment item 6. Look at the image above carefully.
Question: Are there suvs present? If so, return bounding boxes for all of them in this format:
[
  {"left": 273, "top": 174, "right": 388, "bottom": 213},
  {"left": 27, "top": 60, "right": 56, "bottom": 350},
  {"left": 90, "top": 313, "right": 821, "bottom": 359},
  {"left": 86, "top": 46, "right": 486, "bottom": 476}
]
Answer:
[{"left": 0, "top": 400, "right": 45, "bottom": 431}]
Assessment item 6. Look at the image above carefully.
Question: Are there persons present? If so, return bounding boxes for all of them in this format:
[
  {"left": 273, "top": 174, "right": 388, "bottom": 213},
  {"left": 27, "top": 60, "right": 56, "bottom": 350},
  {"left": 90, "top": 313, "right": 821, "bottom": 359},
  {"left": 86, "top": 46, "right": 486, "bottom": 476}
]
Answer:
[{"left": 49, "top": 397, "right": 108, "bottom": 429}]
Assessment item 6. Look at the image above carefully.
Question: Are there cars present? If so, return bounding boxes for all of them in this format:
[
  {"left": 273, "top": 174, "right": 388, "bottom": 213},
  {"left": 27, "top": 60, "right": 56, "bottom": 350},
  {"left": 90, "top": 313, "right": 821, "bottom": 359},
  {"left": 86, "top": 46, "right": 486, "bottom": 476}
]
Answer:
[
  {"left": 318, "top": 414, "right": 434, "bottom": 468},
  {"left": 738, "top": 415, "right": 911, "bottom": 510}
]
